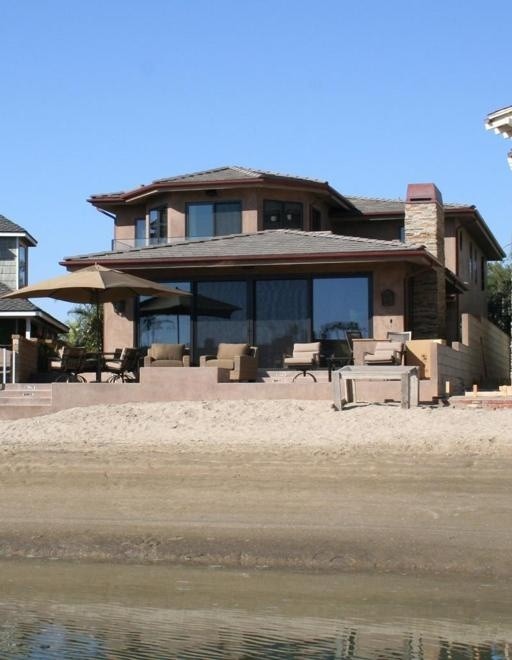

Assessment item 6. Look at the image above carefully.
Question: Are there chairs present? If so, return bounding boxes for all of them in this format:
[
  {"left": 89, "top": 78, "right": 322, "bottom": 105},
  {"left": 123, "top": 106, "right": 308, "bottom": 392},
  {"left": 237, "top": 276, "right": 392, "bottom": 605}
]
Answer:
[
  {"left": 199, "top": 347, "right": 260, "bottom": 383},
  {"left": 47, "top": 346, "right": 140, "bottom": 383},
  {"left": 387, "top": 332, "right": 411, "bottom": 343},
  {"left": 362, "top": 341, "right": 403, "bottom": 365},
  {"left": 345, "top": 331, "right": 362, "bottom": 364},
  {"left": 144, "top": 348, "right": 190, "bottom": 367},
  {"left": 284, "top": 342, "right": 321, "bottom": 382}
]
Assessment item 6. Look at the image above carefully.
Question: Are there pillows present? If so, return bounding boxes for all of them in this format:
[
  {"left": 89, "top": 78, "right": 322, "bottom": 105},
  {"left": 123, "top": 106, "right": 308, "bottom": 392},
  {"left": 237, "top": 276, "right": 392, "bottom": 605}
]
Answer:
[
  {"left": 216, "top": 343, "right": 249, "bottom": 360},
  {"left": 151, "top": 344, "right": 184, "bottom": 360}
]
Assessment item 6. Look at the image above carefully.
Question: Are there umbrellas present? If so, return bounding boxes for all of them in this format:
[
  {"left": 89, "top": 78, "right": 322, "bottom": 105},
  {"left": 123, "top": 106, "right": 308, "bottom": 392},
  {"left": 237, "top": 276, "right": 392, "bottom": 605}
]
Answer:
[
  {"left": 136, "top": 286, "right": 241, "bottom": 344},
  {"left": 0, "top": 261, "right": 194, "bottom": 352}
]
